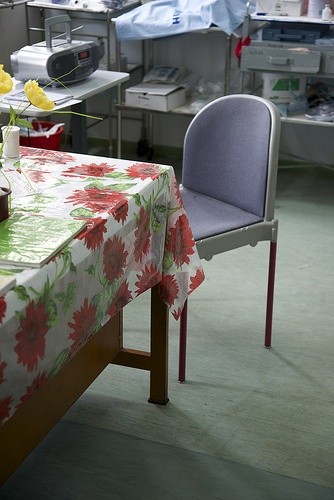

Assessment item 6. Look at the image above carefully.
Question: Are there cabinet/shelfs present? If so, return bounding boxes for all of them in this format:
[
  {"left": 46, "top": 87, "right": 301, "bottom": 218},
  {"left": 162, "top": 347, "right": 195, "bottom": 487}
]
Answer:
[{"left": 23, "top": 0, "right": 334, "bottom": 170}]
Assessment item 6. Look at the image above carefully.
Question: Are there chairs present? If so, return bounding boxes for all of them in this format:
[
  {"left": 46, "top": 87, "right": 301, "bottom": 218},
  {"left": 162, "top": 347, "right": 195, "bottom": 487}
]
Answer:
[{"left": 178, "top": 94, "right": 281, "bottom": 384}]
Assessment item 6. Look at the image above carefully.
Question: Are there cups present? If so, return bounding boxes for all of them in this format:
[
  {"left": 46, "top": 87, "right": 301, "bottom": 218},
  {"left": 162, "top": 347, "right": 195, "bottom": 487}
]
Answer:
[{"left": 1, "top": 125, "right": 20, "bottom": 158}]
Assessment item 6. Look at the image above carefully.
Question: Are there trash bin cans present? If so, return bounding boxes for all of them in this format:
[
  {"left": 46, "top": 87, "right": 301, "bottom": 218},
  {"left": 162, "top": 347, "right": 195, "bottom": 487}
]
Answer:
[{"left": 19, "top": 121, "right": 63, "bottom": 151}]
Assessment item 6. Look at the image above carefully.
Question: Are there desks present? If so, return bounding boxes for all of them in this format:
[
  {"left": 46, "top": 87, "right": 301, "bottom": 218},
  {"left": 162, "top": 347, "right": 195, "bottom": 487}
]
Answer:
[
  {"left": 0, "top": 69, "right": 130, "bottom": 154},
  {"left": 0, "top": 138, "right": 205, "bottom": 490}
]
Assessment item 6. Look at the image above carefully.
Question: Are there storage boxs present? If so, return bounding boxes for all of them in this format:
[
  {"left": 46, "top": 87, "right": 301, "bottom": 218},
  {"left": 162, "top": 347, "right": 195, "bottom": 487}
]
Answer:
[{"left": 125, "top": 82, "right": 185, "bottom": 113}]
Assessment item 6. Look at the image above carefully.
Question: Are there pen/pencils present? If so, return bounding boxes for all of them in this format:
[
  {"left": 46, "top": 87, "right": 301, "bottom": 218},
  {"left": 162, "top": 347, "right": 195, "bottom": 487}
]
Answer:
[
  {"left": 257, "top": 12, "right": 287, "bottom": 15},
  {"left": 55, "top": 97, "right": 71, "bottom": 105}
]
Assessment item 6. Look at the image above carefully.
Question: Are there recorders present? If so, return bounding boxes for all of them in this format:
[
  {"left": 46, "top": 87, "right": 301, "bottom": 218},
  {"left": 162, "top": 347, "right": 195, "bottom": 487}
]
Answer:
[{"left": 9, "top": 14, "right": 102, "bottom": 87}]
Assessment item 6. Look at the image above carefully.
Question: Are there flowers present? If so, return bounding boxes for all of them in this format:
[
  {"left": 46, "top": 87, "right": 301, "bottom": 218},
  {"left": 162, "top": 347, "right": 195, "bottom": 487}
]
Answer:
[{"left": 0, "top": 63, "right": 104, "bottom": 187}]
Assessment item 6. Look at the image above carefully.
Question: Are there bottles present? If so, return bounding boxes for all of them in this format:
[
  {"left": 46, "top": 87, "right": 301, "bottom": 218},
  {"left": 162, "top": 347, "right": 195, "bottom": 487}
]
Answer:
[{"left": 322, "top": 4, "right": 333, "bottom": 20}]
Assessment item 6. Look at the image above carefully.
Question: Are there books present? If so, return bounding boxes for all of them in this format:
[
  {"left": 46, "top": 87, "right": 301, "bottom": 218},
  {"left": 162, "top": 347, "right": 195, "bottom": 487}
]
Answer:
[{"left": 0, "top": 212, "right": 87, "bottom": 268}]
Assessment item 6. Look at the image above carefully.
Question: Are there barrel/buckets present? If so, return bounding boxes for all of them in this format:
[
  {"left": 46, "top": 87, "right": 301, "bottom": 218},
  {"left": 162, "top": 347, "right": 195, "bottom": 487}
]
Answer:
[{"left": 20, "top": 120, "right": 64, "bottom": 151}]
